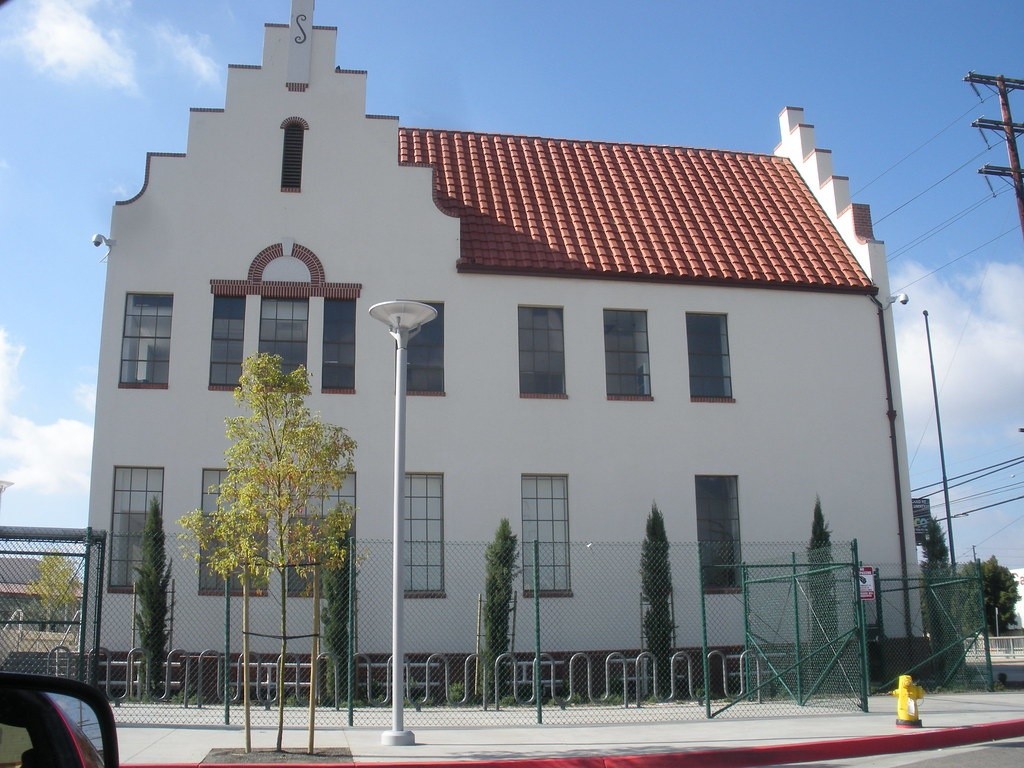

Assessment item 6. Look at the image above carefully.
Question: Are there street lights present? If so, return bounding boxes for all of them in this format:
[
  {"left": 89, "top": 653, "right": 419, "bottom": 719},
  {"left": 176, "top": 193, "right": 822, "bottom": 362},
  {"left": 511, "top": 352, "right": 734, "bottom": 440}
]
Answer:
[{"left": 369, "top": 295, "right": 436, "bottom": 740}]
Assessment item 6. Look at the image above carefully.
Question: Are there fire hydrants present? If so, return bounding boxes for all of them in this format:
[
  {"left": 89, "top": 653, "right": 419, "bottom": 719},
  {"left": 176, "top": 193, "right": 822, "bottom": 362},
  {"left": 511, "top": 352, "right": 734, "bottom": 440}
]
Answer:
[{"left": 893, "top": 675, "right": 924, "bottom": 725}]
